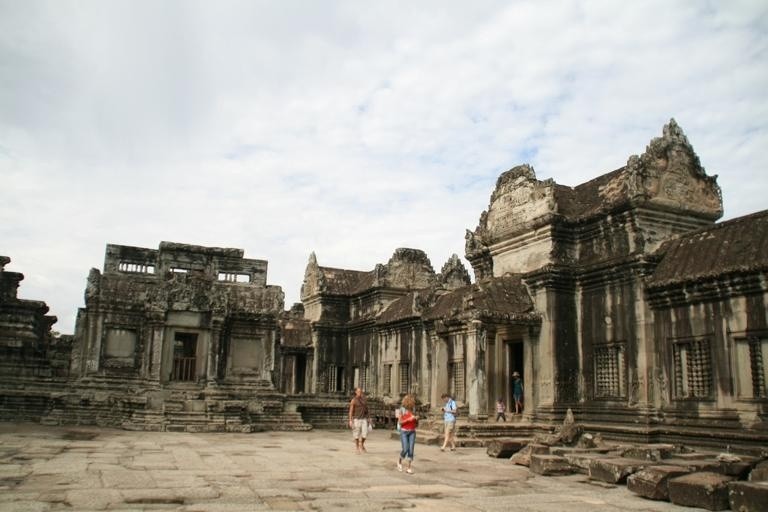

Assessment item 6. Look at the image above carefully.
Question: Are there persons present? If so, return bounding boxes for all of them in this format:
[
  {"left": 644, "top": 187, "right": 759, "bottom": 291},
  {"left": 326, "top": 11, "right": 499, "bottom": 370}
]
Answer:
[
  {"left": 512, "top": 371, "right": 524, "bottom": 415},
  {"left": 496, "top": 398, "right": 506, "bottom": 422},
  {"left": 348, "top": 387, "right": 371, "bottom": 452},
  {"left": 440, "top": 393, "right": 457, "bottom": 451},
  {"left": 396, "top": 395, "right": 420, "bottom": 474}
]
